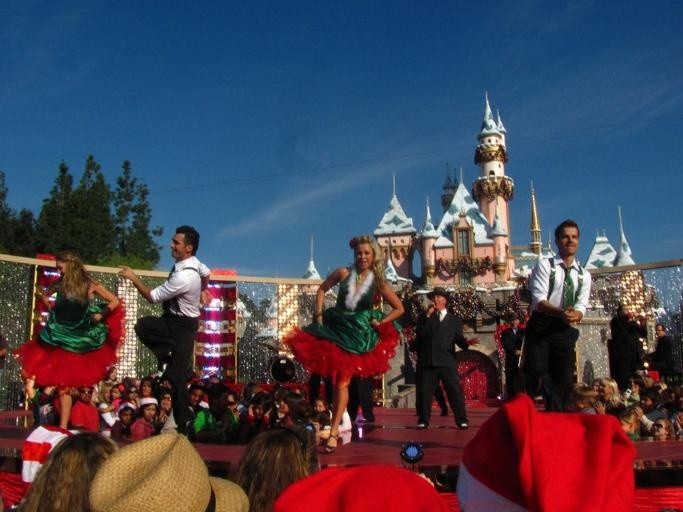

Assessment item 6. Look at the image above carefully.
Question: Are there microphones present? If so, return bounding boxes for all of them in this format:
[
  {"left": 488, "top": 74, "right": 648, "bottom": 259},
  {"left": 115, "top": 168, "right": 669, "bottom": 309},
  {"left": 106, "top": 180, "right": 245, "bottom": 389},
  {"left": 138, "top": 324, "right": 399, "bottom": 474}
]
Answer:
[{"left": 429, "top": 302, "right": 436, "bottom": 308}]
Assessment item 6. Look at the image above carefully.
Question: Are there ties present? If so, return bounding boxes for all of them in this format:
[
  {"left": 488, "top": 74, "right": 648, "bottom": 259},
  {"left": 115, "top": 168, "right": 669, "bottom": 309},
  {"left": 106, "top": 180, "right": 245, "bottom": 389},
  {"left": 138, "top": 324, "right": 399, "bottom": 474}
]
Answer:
[
  {"left": 162, "top": 266, "right": 174, "bottom": 312},
  {"left": 561, "top": 264, "right": 574, "bottom": 310}
]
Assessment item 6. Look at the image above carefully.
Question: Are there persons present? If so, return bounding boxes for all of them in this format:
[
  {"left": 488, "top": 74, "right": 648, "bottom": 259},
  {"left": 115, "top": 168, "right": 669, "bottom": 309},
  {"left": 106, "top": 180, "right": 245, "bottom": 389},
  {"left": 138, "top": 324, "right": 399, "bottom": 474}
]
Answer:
[
  {"left": 567, "top": 303, "right": 683, "bottom": 469},
  {"left": 1, "top": 368, "right": 352, "bottom": 450},
  {"left": 17, "top": 251, "right": 125, "bottom": 429},
  {"left": 118, "top": 224, "right": 211, "bottom": 440},
  {"left": 0, "top": 392, "right": 637, "bottom": 512},
  {"left": 411, "top": 285, "right": 482, "bottom": 430},
  {"left": 283, "top": 235, "right": 406, "bottom": 455},
  {"left": 504, "top": 220, "right": 591, "bottom": 413}
]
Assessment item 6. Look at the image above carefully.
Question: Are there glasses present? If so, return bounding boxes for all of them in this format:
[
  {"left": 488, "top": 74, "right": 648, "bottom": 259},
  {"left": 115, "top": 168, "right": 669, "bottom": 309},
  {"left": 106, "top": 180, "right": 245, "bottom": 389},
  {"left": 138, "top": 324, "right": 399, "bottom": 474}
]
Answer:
[{"left": 652, "top": 424, "right": 665, "bottom": 428}]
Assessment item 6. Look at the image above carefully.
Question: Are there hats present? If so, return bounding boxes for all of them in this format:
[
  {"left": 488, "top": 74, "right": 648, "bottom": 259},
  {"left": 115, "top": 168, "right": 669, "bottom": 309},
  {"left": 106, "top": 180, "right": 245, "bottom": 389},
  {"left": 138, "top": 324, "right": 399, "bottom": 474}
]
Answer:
[
  {"left": 427, "top": 287, "right": 449, "bottom": 299},
  {"left": 274, "top": 465, "right": 450, "bottom": 512},
  {"left": 87, "top": 431, "right": 250, "bottom": 511},
  {"left": 139, "top": 398, "right": 158, "bottom": 405},
  {"left": 118, "top": 397, "right": 136, "bottom": 414},
  {"left": 455, "top": 392, "right": 638, "bottom": 512}
]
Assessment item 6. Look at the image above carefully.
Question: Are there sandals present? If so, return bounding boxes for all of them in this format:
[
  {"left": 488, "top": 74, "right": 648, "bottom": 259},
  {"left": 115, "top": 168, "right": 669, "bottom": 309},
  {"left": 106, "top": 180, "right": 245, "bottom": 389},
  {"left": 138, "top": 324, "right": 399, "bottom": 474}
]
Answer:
[{"left": 324, "top": 433, "right": 338, "bottom": 453}]
